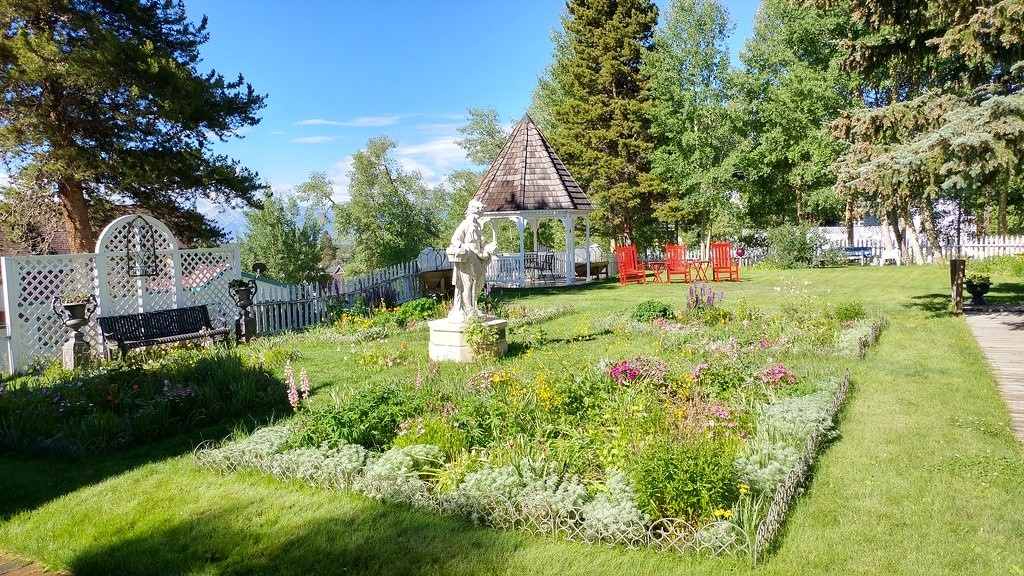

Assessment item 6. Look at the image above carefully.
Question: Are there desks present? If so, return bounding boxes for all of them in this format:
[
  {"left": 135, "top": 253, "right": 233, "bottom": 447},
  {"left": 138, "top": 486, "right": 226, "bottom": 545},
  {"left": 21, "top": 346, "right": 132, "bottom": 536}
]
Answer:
[
  {"left": 651, "top": 261, "right": 666, "bottom": 284},
  {"left": 692, "top": 259, "right": 710, "bottom": 283}
]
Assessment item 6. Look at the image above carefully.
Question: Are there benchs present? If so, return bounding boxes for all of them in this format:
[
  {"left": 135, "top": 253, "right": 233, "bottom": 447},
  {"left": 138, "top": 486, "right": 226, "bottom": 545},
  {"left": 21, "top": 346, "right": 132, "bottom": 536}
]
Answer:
[
  {"left": 96, "top": 303, "right": 231, "bottom": 366},
  {"left": 819, "top": 247, "right": 873, "bottom": 266}
]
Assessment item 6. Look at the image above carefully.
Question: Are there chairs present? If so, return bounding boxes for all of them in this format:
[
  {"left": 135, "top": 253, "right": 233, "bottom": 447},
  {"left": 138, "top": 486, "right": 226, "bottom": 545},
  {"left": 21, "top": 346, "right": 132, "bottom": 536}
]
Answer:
[
  {"left": 712, "top": 241, "right": 740, "bottom": 284},
  {"left": 524, "top": 253, "right": 556, "bottom": 283},
  {"left": 666, "top": 241, "right": 693, "bottom": 284},
  {"left": 617, "top": 244, "right": 646, "bottom": 285}
]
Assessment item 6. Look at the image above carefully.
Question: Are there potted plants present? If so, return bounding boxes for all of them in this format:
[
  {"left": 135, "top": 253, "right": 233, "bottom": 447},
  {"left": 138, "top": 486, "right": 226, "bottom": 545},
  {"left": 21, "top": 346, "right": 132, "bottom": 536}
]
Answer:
[{"left": 966, "top": 274, "right": 991, "bottom": 307}]
